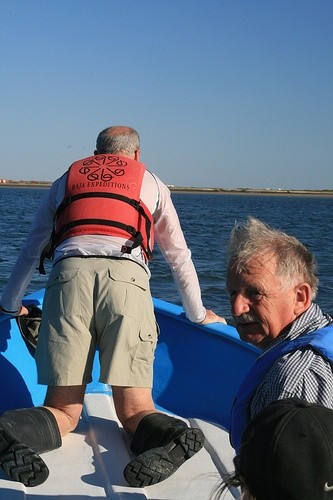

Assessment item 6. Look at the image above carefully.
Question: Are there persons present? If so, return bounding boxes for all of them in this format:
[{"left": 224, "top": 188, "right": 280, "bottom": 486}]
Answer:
[
  {"left": 2, "top": 127, "right": 225, "bottom": 487},
  {"left": 226, "top": 216, "right": 333, "bottom": 500}
]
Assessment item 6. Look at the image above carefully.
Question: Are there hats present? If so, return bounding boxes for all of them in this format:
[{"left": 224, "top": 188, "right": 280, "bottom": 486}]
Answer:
[{"left": 235, "top": 399, "right": 333, "bottom": 500}]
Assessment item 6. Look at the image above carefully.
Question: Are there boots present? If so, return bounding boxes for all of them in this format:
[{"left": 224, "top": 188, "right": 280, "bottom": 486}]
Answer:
[
  {"left": 123, "top": 412, "right": 204, "bottom": 489},
  {"left": 0, "top": 406, "right": 62, "bottom": 487}
]
